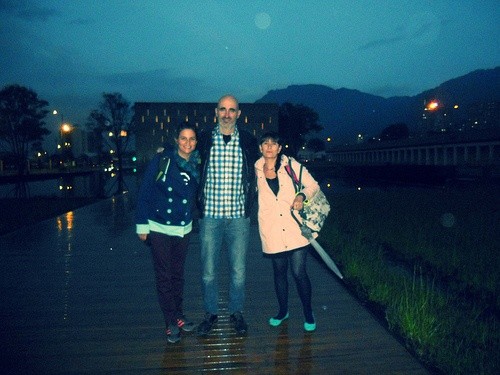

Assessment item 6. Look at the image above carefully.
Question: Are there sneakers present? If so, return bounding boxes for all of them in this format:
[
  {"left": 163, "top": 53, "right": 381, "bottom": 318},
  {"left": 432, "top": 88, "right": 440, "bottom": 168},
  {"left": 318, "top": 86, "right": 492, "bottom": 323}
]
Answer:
[
  {"left": 176, "top": 316, "right": 194, "bottom": 331},
  {"left": 197, "top": 313, "right": 218, "bottom": 336},
  {"left": 166, "top": 324, "right": 182, "bottom": 344},
  {"left": 230, "top": 311, "right": 247, "bottom": 335}
]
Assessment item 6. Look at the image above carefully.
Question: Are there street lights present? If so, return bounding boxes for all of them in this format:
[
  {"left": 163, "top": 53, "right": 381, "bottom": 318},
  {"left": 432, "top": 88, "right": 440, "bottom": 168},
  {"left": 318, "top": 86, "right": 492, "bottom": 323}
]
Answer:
[{"left": 53, "top": 110, "right": 63, "bottom": 149}]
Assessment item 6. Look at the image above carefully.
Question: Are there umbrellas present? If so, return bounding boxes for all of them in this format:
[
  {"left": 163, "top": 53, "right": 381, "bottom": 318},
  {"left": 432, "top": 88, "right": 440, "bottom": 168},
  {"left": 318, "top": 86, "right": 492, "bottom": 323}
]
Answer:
[{"left": 291, "top": 206, "right": 347, "bottom": 284}]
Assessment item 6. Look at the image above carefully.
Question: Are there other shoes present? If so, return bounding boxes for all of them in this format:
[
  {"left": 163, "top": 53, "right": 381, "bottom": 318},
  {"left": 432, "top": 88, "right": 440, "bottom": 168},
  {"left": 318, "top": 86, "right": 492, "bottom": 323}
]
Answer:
[
  {"left": 269, "top": 311, "right": 289, "bottom": 326},
  {"left": 304, "top": 311, "right": 316, "bottom": 331}
]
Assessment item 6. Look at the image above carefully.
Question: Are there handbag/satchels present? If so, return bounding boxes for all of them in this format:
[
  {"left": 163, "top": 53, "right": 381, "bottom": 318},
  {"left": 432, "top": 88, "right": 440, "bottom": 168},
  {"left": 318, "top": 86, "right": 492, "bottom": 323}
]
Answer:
[
  {"left": 145, "top": 155, "right": 170, "bottom": 245},
  {"left": 289, "top": 157, "right": 331, "bottom": 232}
]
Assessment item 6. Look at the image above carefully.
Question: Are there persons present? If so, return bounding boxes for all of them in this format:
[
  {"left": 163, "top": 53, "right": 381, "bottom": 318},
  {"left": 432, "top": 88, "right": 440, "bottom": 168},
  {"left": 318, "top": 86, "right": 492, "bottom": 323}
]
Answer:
[
  {"left": 254, "top": 130, "right": 320, "bottom": 332},
  {"left": 135, "top": 120, "right": 201, "bottom": 344},
  {"left": 157, "top": 95, "right": 262, "bottom": 335}
]
